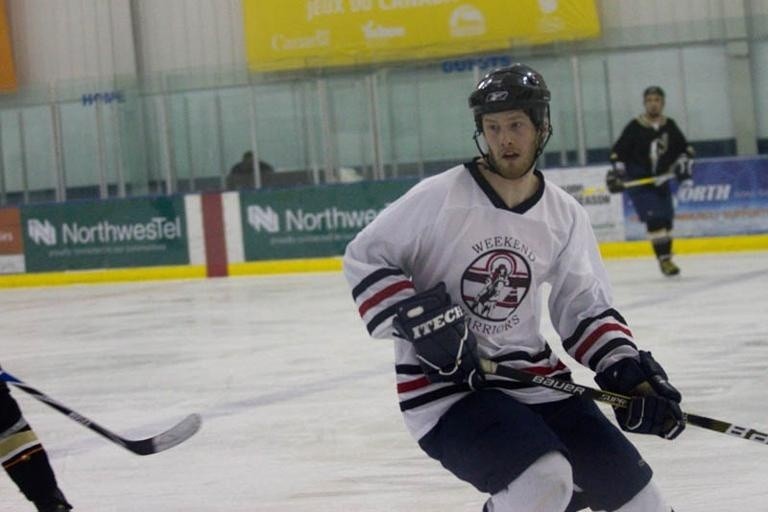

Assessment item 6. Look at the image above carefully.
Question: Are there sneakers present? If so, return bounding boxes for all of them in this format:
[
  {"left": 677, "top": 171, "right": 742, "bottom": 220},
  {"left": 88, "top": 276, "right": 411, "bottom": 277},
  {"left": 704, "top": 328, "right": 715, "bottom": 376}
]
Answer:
[{"left": 659, "top": 260, "right": 681, "bottom": 276}]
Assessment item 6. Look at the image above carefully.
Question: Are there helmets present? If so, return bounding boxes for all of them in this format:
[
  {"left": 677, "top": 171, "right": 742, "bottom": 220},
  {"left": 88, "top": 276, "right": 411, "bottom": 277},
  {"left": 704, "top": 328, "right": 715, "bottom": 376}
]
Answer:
[
  {"left": 642, "top": 84, "right": 667, "bottom": 98},
  {"left": 467, "top": 64, "right": 552, "bottom": 132}
]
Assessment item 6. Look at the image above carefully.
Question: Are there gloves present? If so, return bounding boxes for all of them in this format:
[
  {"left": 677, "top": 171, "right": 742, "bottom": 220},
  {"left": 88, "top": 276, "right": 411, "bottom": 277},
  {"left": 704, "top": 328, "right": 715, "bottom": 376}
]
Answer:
[
  {"left": 605, "top": 166, "right": 629, "bottom": 194},
  {"left": 671, "top": 157, "right": 695, "bottom": 180},
  {"left": 393, "top": 281, "right": 489, "bottom": 391},
  {"left": 593, "top": 349, "right": 686, "bottom": 440}
]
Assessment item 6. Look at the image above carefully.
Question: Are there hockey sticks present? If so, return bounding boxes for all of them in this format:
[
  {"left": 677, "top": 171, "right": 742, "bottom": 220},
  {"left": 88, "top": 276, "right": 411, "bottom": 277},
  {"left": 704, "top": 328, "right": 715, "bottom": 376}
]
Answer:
[{"left": 0, "top": 367, "right": 201, "bottom": 455}]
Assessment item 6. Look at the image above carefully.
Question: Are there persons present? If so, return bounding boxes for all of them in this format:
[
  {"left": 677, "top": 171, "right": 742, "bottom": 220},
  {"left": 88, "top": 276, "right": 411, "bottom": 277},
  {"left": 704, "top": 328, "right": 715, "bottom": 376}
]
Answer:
[
  {"left": 0, "top": 374, "right": 73, "bottom": 511},
  {"left": 342, "top": 61, "right": 686, "bottom": 511},
  {"left": 608, "top": 85, "right": 696, "bottom": 276},
  {"left": 225, "top": 151, "right": 276, "bottom": 190}
]
